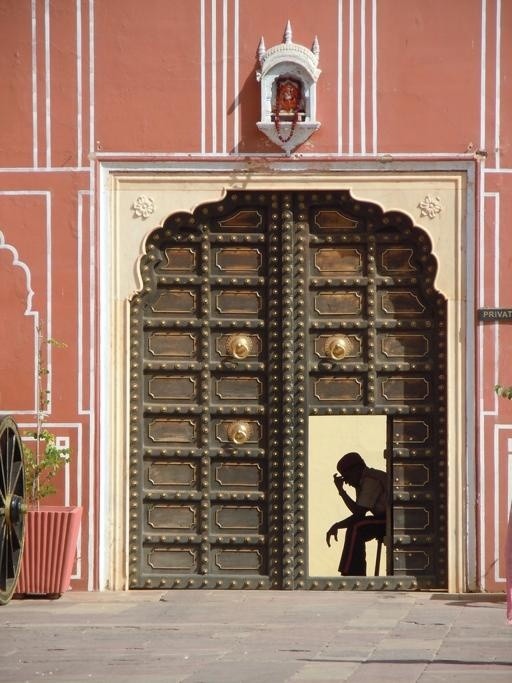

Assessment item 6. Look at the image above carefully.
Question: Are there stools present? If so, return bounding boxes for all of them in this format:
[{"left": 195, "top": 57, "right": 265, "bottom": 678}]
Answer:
[{"left": 374, "top": 536, "right": 386, "bottom": 575}]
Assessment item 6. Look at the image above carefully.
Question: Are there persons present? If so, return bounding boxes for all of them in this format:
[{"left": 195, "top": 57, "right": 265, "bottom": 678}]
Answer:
[{"left": 326, "top": 453, "right": 387, "bottom": 576}]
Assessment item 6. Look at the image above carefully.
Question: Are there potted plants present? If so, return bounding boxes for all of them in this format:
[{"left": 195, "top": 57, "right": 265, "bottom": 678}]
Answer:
[{"left": 16, "top": 323, "right": 83, "bottom": 599}]
